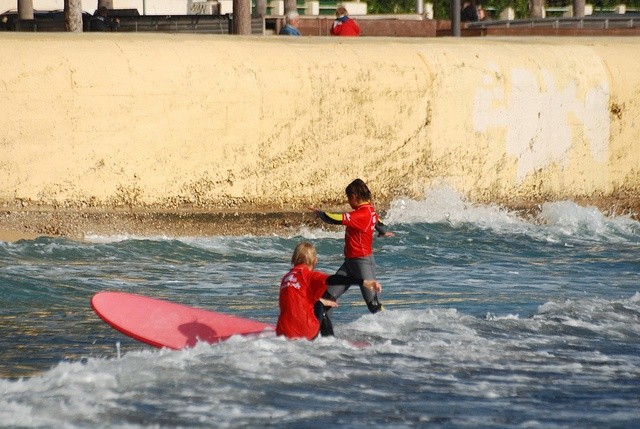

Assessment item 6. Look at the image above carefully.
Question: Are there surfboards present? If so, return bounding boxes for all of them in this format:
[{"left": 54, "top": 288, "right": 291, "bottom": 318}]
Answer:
[{"left": 91, "top": 292, "right": 371, "bottom": 351}]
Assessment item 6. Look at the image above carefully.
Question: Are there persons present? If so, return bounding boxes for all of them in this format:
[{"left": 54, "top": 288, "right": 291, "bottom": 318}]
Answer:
[
  {"left": 308, "top": 179, "right": 396, "bottom": 314},
  {"left": 0, "top": 15, "right": 8, "bottom": 31},
  {"left": 476, "top": 9, "right": 492, "bottom": 20},
  {"left": 461, "top": 2, "right": 477, "bottom": 22},
  {"left": 275, "top": 243, "right": 340, "bottom": 342},
  {"left": 91, "top": 7, "right": 119, "bottom": 32},
  {"left": 279, "top": 11, "right": 303, "bottom": 36},
  {"left": 330, "top": 8, "right": 361, "bottom": 37}
]
